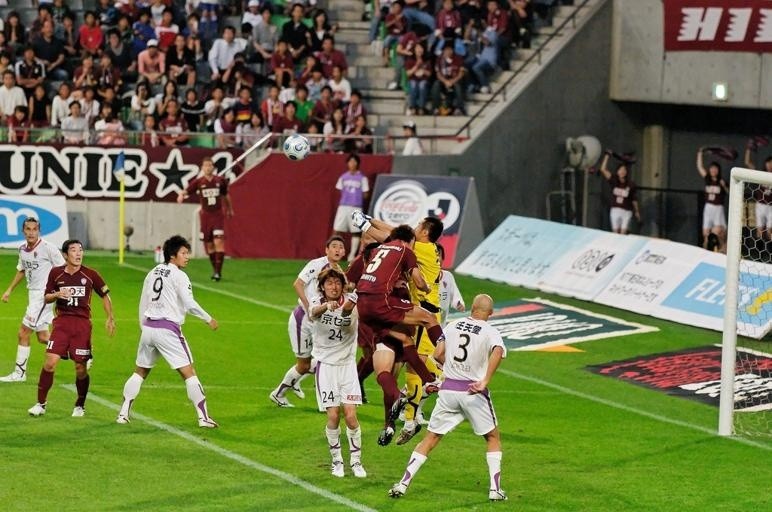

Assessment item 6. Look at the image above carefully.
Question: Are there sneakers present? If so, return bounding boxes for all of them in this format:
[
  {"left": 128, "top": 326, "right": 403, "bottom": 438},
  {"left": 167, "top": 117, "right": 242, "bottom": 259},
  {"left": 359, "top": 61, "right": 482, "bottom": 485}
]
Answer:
[
  {"left": 269, "top": 391, "right": 296, "bottom": 408},
  {"left": 290, "top": 385, "right": 305, "bottom": 399},
  {"left": 198, "top": 416, "right": 219, "bottom": 428},
  {"left": 331, "top": 457, "right": 345, "bottom": 477},
  {"left": 489, "top": 489, "right": 508, "bottom": 501},
  {"left": 422, "top": 372, "right": 443, "bottom": 399},
  {"left": 349, "top": 457, "right": 367, "bottom": 478},
  {"left": 388, "top": 483, "right": 407, "bottom": 498},
  {"left": 28, "top": 401, "right": 48, "bottom": 415},
  {"left": 377, "top": 392, "right": 429, "bottom": 444},
  {"left": 116, "top": 415, "right": 130, "bottom": 424},
  {"left": 71, "top": 406, "right": 85, "bottom": 417},
  {"left": 211, "top": 272, "right": 220, "bottom": 282},
  {"left": 0, "top": 372, "right": 26, "bottom": 382}
]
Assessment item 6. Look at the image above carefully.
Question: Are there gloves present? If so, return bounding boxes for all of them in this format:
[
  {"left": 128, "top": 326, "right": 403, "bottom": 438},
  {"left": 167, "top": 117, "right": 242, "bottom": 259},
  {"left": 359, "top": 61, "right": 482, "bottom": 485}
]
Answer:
[
  {"left": 346, "top": 289, "right": 358, "bottom": 304},
  {"left": 351, "top": 210, "right": 372, "bottom": 233}
]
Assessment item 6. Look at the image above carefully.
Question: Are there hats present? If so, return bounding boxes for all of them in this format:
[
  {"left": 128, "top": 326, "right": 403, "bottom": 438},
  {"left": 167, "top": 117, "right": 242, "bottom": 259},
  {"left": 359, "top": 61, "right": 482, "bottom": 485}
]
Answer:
[
  {"left": 248, "top": 0, "right": 259, "bottom": 8},
  {"left": 402, "top": 120, "right": 416, "bottom": 129},
  {"left": 146, "top": 39, "right": 159, "bottom": 48}
]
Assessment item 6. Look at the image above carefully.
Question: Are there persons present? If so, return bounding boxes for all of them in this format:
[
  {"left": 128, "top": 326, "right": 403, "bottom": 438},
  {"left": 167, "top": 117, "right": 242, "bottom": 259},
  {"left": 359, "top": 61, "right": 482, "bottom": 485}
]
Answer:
[
  {"left": 600, "top": 137, "right": 771, "bottom": 259},
  {"left": 0, "top": 1, "right": 565, "bottom": 283},
  {"left": 27, "top": 240, "right": 115, "bottom": 417},
  {"left": 269, "top": 210, "right": 508, "bottom": 501},
  {"left": 0, "top": 217, "right": 67, "bottom": 381},
  {"left": 116, "top": 234, "right": 221, "bottom": 428}
]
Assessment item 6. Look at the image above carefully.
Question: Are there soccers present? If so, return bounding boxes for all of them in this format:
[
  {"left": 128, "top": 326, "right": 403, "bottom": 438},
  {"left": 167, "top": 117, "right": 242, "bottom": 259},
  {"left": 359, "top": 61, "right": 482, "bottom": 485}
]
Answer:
[{"left": 283, "top": 135, "right": 313, "bottom": 163}]
